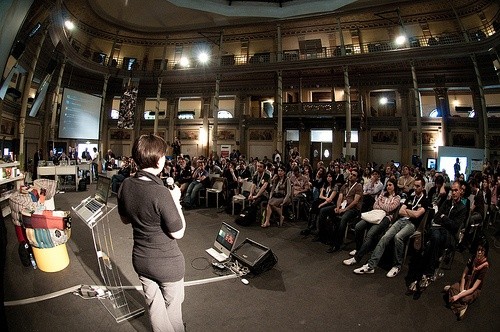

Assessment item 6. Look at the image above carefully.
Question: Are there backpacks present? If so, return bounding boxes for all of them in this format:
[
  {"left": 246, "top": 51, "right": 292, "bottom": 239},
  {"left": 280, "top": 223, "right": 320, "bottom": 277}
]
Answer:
[
  {"left": 235, "top": 204, "right": 257, "bottom": 226},
  {"left": 78, "top": 178, "right": 87, "bottom": 191}
]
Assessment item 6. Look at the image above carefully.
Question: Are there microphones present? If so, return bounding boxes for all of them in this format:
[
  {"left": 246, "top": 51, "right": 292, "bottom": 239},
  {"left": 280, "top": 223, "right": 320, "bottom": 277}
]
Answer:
[{"left": 166, "top": 177, "right": 175, "bottom": 190}]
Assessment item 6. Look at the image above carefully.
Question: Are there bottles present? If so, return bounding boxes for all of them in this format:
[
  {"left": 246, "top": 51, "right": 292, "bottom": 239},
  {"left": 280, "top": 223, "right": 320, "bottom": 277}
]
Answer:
[{"left": 30, "top": 254, "right": 37, "bottom": 269}]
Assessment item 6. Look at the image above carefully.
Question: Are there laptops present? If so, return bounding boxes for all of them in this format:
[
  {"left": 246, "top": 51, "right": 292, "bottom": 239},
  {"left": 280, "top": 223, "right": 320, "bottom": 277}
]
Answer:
[{"left": 77, "top": 174, "right": 111, "bottom": 221}]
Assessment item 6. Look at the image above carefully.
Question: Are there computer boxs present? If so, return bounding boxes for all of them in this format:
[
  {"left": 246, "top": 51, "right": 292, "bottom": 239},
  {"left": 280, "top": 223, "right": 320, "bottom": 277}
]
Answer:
[{"left": 205, "top": 248, "right": 227, "bottom": 263}]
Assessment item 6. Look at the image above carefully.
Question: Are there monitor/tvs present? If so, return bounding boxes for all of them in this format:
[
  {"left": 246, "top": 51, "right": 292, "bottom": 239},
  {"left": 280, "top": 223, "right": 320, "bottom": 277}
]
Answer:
[
  {"left": 426, "top": 158, "right": 437, "bottom": 170},
  {"left": 213, "top": 222, "right": 240, "bottom": 257}
]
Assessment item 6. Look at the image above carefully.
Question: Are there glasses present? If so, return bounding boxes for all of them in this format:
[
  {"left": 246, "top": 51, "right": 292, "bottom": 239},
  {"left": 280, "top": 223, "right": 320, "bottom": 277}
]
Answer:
[{"left": 476, "top": 248, "right": 486, "bottom": 252}]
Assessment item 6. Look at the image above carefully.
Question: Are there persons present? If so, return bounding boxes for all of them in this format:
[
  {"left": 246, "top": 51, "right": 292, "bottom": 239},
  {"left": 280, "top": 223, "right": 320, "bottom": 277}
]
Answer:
[
  {"left": 272, "top": 150, "right": 280, "bottom": 163},
  {"left": 160, "top": 151, "right": 313, "bottom": 227},
  {"left": 289, "top": 147, "right": 300, "bottom": 165},
  {"left": 69, "top": 147, "right": 78, "bottom": 159},
  {"left": 339, "top": 153, "right": 346, "bottom": 163},
  {"left": 454, "top": 158, "right": 460, "bottom": 181},
  {"left": 301, "top": 160, "right": 500, "bottom": 320},
  {"left": 105, "top": 150, "right": 139, "bottom": 196},
  {"left": 172, "top": 136, "right": 181, "bottom": 157},
  {"left": 117, "top": 134, "right": 185, "bottom": 332},
  {"left": 82, "top": 148, "right": 99, "bottom": 181},
  {"left": 230, "top": 149, "right": 235, "bottom": 160}
]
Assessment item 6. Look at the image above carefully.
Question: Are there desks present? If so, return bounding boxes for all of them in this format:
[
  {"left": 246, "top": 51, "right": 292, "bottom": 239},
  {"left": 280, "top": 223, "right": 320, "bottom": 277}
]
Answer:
[
  {"left": 37, "top": 165, "right": 79, "bottom": 192},
  {"left": 78, "top": 161, "right": 93, "bottom": 184}
]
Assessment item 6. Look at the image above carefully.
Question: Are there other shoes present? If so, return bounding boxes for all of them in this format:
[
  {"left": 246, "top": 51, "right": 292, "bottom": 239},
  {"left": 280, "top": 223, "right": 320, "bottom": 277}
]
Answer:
[
  {"left": 94, "top": 177, "right": 98, "bottom": 181},
  {"left": 443, "top": 285, "right": 451, "bottom": 292},
  {"left": 300, "top": 227, "right": 312, "bottom": 235},
  {"left": 343, "top": 257, "right": 359, "bottom": 266},
  {"left": 326, "top": 244, "right": 339, "bottom": 253},
  {"left": 261, "top": 222, "right": 270, "bottom": 227},
  {"left": 311, "top": 236, "right": 322, "bottom": 242},
  {"left": 459, "top": 304, "right": 468, "bottom": 320},
  {"left": 406, "top": 274, "right": 431, "bottom": 296},
  {"left": 111, "top": 192, "right": 117, "bottom": 194},
  {"left": 279, "top": 216, "right": 285, "bottom": 228},
  {"left": 350, "top": 250, "right": 357, "bottom": 255}
]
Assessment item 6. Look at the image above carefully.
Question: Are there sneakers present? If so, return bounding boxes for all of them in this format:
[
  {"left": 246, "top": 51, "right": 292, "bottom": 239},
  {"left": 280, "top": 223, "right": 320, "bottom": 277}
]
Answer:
[
  {"left": 353, "top": 264, "right": 375, "bottom": 275},
  {"left": 387, "top": 266, "right": 401, "bottom": 277}
]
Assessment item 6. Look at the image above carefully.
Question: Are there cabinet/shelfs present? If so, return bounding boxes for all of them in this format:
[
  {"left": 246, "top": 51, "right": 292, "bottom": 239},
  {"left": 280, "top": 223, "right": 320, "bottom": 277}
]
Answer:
[{"left": 0, "top": 162, "right": 26, "bottom": 217}]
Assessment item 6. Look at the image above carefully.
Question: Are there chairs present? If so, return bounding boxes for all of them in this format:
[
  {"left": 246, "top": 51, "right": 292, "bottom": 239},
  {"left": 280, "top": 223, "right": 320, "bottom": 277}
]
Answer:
[
  {"left": 20, "top": 208, "right": 71, "bottom": 272},
  {"left": 333, "top": 206, "right": 498, "bottom": 281},
  {"left": 194, "top": 173, "right": 300, "bottom": 223},
  {"left": 21, "top": 178, "right": 57, "bottom": 215},
  {"left": 9, "top": 193, "right": 41, "bottom": 265}
]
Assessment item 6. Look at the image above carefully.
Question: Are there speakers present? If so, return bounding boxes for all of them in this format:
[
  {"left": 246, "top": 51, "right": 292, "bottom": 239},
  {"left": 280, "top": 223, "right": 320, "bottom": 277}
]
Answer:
[{"left": 230, "top": 237, "right": 276, "bottom": 275}]
